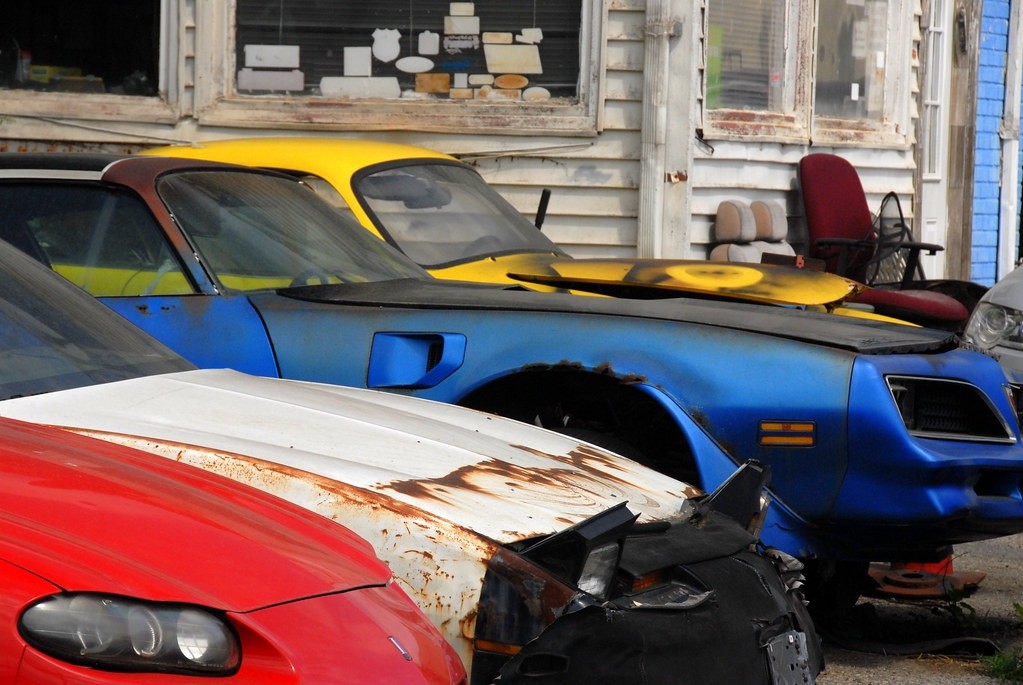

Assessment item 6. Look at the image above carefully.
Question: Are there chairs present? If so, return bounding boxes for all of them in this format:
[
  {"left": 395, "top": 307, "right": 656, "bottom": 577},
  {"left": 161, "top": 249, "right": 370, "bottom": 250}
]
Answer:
[
  {"left": 750, "top": 200, "right": 795, "bottom": 257},
  {"left": 710, "top": 199, "right": 762, "bottom": 264},
  {"left": 796, "top": 153, "right": 969, "bottom": 329},
  {"left": 54, "top": 197, "right": 151, "bottom": 265}
]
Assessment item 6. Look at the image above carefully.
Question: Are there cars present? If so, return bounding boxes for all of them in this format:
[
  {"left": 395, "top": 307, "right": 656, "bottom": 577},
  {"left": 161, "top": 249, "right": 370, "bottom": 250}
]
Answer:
[
  {"left": 0, "top": 236, "right": 827, "bottom": 684},
  {"left": 0, "top": 135, "right": 1022, "bottom": 611}
]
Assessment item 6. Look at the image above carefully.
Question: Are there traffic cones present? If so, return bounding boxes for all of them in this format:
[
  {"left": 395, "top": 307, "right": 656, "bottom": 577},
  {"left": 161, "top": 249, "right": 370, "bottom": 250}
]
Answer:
[{"left": 868, "top": 546, "right": 987, "bottom": 599}]
